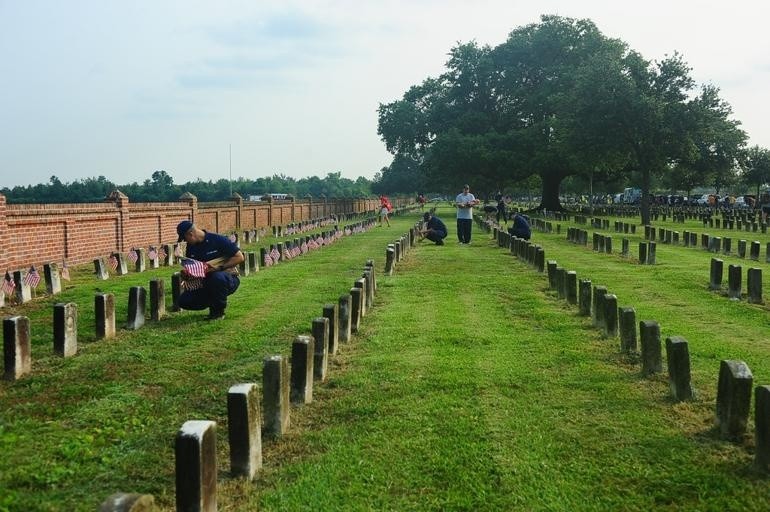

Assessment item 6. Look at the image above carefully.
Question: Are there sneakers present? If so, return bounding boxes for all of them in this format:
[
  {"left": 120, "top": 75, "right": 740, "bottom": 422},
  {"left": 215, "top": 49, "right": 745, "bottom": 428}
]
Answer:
[{"left": 203, "top": 308, "right": 227, "bottom": 324}]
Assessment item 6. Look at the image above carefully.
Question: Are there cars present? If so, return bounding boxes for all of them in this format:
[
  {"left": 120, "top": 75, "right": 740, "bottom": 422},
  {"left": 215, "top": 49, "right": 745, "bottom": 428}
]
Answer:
[{"left": 560, "top": 188, "right": 749, "bottom": 208}]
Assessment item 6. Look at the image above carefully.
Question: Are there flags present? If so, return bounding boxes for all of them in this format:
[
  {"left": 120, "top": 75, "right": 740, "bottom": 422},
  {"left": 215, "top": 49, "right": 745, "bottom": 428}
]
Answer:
[
  {"left": 0, "top": 269, "right": 16, "bottom": 297},
  {"left": 103, "top": 216, "right": 376, "bottom": 272},
  {"left": 179, "top": 256, "right": 206, "bottom": 278},
  {"left": 181, "top": 269, "right": 204, "bottom": 292},
  {"left": 24, "top": 263, "right": 40, "bottom": 289},
  {"left": 61, "top": 257, "right": 71, "bottom": 281}
]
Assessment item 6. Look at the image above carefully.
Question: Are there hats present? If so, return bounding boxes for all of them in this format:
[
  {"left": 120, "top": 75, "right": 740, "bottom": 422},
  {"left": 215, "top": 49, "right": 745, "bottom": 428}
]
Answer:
[{"left": 175, "top": 219, "right": 194, "bottom": 242}]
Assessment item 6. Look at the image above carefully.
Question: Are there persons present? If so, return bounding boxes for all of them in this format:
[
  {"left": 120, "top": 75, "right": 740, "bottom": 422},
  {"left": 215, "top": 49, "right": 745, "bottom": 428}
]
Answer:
[
  {"left": 494, "top": 189, "right": 508, "bottom": 224},
  {"left": 653, "top": 193, "right": 737, "bottom": 210},
  {"left": 176, "top": 220, "right": 246, "bottom": 323},
  {"left": 415, "top": 192, "right": 426, "bottom": 212},
  {"left": 418, "top": 213, "right": 448, "bottom": 246},
  {"left": 455, "top": 184, "right": 475, "bottom": 245},
  {"left": 482, "top": 203, "right": 498, "bottom": 223},
  {"left": 508, "top": 211, "right": 531, "bottom": 241},
  {"left": 376, "top": 194, "right": 392, "bottom": 228},
  {"left": 761, "top": 181, "right": 770, "bottom": 224}
]
